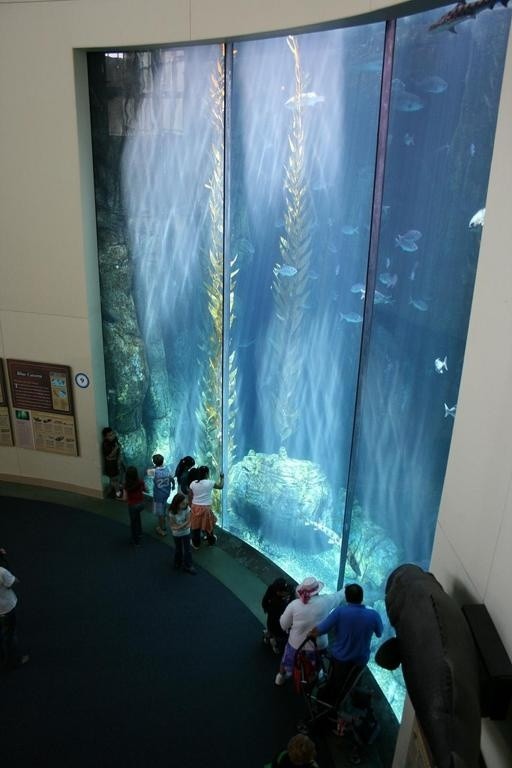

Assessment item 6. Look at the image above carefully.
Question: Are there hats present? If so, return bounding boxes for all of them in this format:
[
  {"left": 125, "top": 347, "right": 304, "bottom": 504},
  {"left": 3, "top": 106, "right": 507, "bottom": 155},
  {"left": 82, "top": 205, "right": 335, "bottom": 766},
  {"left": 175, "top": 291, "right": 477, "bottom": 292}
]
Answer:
[{"left": 294, "top": 576, "right": 325, "bottom": 600}]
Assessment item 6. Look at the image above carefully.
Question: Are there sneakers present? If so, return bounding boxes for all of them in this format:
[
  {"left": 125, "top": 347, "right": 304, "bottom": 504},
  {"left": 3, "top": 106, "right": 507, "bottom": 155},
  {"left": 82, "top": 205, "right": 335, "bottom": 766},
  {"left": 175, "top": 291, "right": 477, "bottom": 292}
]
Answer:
[
  {"left": 189, "top": 534, "right": 218, "bottom": 552},
  {"left": 173, "top": 565, "right": 198, "bottom": 576},
  {"left": 275, "top": 671, "right": 292, "bottom": 686}
]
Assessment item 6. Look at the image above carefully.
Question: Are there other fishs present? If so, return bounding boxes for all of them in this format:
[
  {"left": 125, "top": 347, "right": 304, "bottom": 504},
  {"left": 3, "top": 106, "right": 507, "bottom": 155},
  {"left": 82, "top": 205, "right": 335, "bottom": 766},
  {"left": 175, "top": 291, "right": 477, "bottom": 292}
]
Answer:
[
  {"left": 374, "top": 556, "right": 498, "bottom": 767},
  {"left": 424, "top": 1, "right": 511, "bottom": 41},
  {"left": 234, "top": 71, "right": 451, "bottom": 312}
]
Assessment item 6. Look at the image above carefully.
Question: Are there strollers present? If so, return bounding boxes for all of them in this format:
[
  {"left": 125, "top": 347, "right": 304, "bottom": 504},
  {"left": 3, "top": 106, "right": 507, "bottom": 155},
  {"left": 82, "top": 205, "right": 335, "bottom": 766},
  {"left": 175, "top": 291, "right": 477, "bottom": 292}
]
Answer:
[
  {"left": 295, "top": 634, "right": 382, "bottom": 765},
  {"left": 261, "top": 576, "right": 297, "bottom": 655}
]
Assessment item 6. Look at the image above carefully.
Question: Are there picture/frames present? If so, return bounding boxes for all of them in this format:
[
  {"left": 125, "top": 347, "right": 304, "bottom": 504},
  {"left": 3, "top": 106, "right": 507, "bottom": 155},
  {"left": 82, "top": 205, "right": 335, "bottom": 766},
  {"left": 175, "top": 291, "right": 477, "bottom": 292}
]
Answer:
[{"left": 0, "top": 358, "right": 78, "bottom": 457}]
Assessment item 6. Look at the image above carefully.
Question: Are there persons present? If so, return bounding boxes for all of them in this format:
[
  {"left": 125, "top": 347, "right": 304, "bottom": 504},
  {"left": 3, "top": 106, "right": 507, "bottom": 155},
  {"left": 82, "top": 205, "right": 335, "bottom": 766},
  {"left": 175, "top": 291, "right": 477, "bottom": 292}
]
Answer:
[
  {"left": 147, "top": 454, "right": 175, "bottom": 537},
  {"left": 168, "top": 493, "right": 198, "bottom": 575},
  {"left": 173, "top": 456, "right": 196, "bottom": 502},
  {"left": 189, "top": 465, "right": 225, "bottom": 549},
  {"left": 269, "top": 732, "right": 320, "bottom": 768},
  {"left": 125, "top": 465, "right": 150, "bottom": 545},
  {"left": 186, "top": 467, "right": 208, "bottom": 541},
  {"left": 0, "top": 548, "right": 32, "bottom": 666},
  {"left": 306, "top": 582, "right": 384, "bottom": 724},
  {"left": 261, "top": 576, "right": 289, "bottom": 640},
  {"left": 274, "top": 576, "right": 348, "bottom": 685},
  {"left": 100, "top": 426, "right": 123, "bottom": 498}
]
